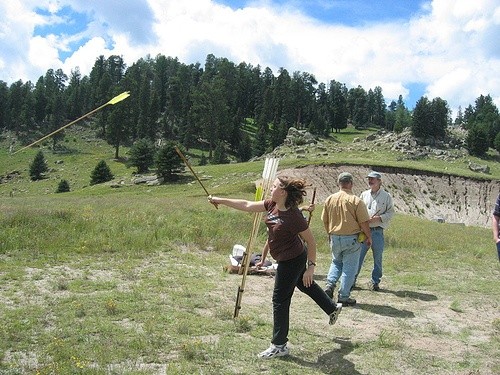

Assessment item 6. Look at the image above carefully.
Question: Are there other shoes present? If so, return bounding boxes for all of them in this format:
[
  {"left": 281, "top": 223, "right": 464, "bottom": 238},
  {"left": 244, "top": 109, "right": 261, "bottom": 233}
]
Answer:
[
  {"left": 373, "top": 283, "right": 379, "bottom": 290},
  {"left": 337, "top": 298, "right": 355, "bottom": 306},
  {"left": 324, "top": 287, "right": 333, "bottom": 298}
]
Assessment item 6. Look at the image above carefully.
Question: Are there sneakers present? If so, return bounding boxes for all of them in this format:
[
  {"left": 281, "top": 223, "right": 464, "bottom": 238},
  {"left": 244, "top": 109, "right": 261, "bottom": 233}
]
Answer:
[
  {"left": 257, "top": 343, "right": 288, "bottom": 358},
  {"left": 328, "top": 302, "right": 343, "bottom": 325}
]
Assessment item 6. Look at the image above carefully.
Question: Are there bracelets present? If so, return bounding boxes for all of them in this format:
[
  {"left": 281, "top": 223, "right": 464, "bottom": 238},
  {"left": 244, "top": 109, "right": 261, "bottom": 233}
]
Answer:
[{"left": 308, "top": 260, "right": 316, "bottom": 266}]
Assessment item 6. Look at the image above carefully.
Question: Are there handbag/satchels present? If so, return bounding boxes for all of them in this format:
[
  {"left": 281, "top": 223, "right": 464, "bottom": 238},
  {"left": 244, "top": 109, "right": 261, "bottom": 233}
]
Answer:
[{"left": 358, "top": 230, "right": 366, "bottom": 242}]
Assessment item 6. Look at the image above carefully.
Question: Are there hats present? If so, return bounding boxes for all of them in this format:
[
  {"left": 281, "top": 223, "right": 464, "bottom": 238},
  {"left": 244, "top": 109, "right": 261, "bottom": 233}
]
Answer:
[{"left": 365, "top": 171, "right": 381, "bottom": 178}]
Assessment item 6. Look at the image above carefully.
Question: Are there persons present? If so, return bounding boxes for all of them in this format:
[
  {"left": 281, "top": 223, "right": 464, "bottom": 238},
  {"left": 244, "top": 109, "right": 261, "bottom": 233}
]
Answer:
[
  {"left": 352, "top": 171, "right": 394, "bottom": 291},
  {"left": 492, "top": 194, "right": 500, "bottom": 261},
  {"left": 321, "top": 172, "right": 372, "bottom": 306},
  {"left": 255, "top": 203, "right": 315, "bottom": 267},
  {"left": 207, "top": 176, "right": 342, "bottom": 359}
]
